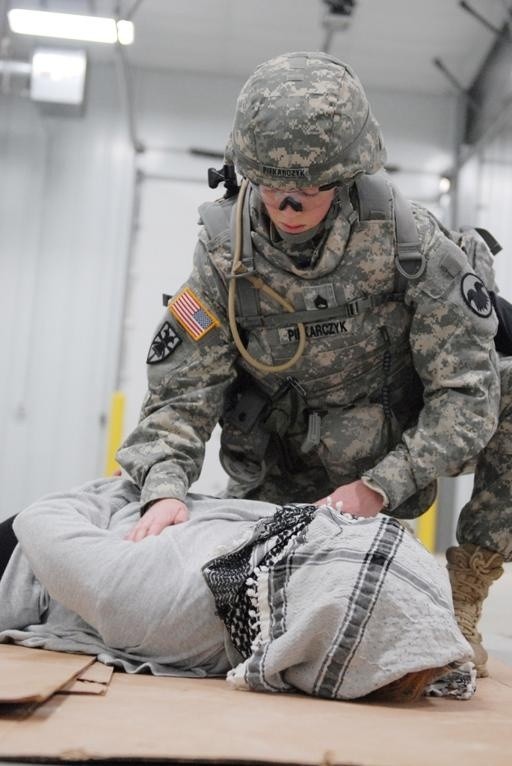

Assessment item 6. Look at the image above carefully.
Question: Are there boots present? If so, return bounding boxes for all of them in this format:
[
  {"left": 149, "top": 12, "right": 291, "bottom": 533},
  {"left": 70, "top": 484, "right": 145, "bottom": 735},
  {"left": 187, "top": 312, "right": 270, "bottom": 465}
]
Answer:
[{"left": 446, "top": 543, "right": 504, "bottom": 679}]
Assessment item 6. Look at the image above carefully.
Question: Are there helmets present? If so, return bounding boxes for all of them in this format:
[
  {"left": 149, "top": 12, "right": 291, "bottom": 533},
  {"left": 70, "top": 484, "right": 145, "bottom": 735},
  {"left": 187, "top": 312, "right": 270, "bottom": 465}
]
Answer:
[{"left": 208, "top": 51, "right": 385, "bottom": 193}]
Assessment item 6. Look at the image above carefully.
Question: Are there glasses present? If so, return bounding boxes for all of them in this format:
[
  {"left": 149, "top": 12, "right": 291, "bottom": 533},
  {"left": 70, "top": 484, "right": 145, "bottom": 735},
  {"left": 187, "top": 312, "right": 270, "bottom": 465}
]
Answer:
[{"left": 247, "top": 180, "right": 339, "bottom": 207}]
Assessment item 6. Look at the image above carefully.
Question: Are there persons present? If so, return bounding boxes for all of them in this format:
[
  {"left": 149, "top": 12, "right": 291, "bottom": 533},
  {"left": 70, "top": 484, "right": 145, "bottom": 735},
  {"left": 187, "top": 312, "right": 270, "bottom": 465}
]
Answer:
[
  {"left": 415, "top": 215, "right": 512, "bottom": 702},
  {"left": 108, "top": 49, "right": 503, "bottom": 540},
  {"left": 3, "top": 474, "right": 475, "bottom": 710}
]
